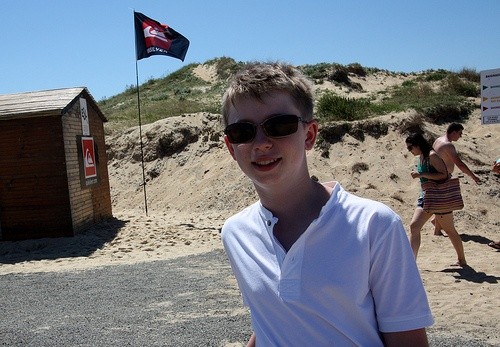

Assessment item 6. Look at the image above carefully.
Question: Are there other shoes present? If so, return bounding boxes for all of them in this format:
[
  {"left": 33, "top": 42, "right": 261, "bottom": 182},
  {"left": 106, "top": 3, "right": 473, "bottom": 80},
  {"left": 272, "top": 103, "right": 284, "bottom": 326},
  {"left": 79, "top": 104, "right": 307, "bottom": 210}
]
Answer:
[
  {"left": 451, "top": 261, "right": 467, "bottom": 266},
  {"left": 431, "top": 219, "right": 436, "bottom": 226},
  {"left": 434, "top": 230, "right": 443, "bottom": 235}
]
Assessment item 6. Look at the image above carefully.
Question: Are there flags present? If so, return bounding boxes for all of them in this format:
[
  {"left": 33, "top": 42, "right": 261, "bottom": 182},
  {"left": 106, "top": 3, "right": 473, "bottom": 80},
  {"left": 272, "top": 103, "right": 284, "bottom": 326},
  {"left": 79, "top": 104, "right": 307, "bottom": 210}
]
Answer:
[{"left": 133, "top": 9, "right": 190, "bottom": 62}]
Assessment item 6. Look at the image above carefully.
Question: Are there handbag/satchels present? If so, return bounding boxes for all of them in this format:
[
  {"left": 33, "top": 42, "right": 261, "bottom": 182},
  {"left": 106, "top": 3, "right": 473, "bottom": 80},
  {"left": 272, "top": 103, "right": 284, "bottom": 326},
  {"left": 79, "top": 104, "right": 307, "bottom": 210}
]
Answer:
[{"left": 421, "top": 178, "right": 464, "bottom": 213}]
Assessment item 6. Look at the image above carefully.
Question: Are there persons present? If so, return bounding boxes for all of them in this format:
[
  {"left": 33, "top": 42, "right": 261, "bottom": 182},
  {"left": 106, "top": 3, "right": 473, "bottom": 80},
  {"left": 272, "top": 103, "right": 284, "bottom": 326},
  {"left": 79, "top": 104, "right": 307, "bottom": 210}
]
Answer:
[
  {"left": 405, "top": 133, "right": 467, "bottom": 267},
  {"left": 488, "top": 159, "right": 500, "bottom": 249},
  {"left": 220, "top": 60, "right": 435, "bottom": 347},
  {"left": 432, "top": 123, "right": 481, "bottom": 236}
]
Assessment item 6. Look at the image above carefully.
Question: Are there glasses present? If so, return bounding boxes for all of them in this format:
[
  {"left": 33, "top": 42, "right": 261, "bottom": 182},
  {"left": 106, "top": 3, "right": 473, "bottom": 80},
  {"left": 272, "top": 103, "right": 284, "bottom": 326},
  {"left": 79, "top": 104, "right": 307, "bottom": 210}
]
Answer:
[
  {"left": 224, "top": 113, "right": 310, "bottom": 144},
  {"left": 408, "top": 144, "right": 415, "bottom": 151}
]
